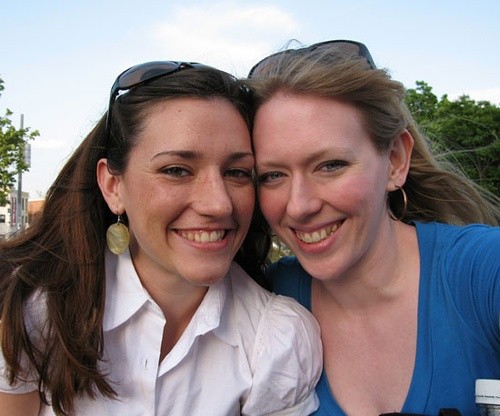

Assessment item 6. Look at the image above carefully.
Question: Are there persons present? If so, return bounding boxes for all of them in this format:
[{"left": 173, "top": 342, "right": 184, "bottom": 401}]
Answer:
[
  {"left": 246, "top": 39, "right": 500, "bottom": 416},
  {"left": 0, "top": 61, "right": 324, "bottom": 416}
]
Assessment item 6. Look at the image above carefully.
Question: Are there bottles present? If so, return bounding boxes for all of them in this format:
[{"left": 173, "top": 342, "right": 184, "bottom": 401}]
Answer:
[{"left": 474, "top": 378, "right": 500, "bottom": 416}]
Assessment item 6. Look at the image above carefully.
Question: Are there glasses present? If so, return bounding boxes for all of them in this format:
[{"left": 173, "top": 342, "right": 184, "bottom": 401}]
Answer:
[
  {"left": 103, "top": 60, "right": 208, "bottom": 156},
  {"left": 248, "top": 39, "right": 377, "bottom": 81}
]
